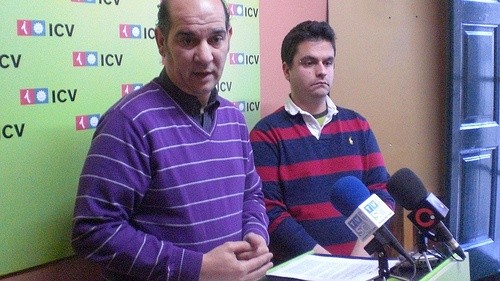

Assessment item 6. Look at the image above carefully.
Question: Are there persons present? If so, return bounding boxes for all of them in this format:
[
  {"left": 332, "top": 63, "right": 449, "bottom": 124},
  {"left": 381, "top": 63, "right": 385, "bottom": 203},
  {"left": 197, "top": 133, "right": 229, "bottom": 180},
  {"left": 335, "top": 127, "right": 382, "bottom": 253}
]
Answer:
[
  {"left": 68, "top": 0, "right": 274, "bottom": 281},
  {"left": 249, "top": 20, "right": 396, "bottom": 268}
]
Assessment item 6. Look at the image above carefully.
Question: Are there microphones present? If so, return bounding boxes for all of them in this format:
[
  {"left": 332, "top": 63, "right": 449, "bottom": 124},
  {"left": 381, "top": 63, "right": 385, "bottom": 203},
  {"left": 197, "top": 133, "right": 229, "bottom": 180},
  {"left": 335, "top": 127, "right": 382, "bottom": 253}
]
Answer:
[
  {"left": 331, "top": 175, "right": 417, "bottom": 266},
  {"left": 385, "top": 167, "right": 467, "bottom": 260}
]
